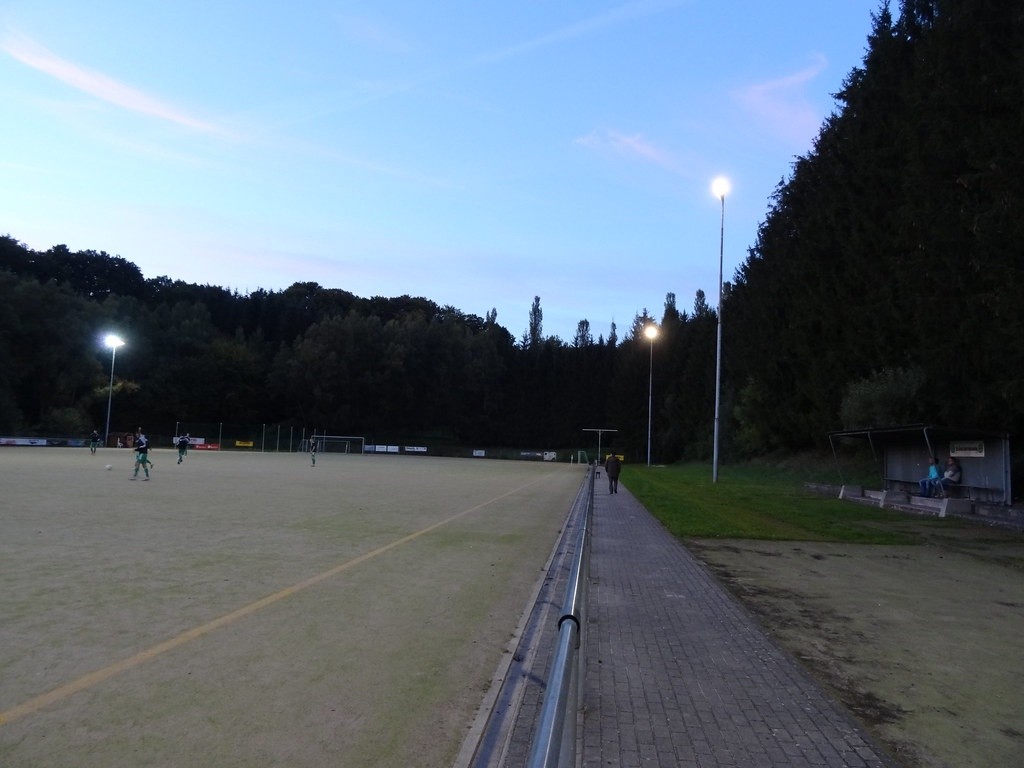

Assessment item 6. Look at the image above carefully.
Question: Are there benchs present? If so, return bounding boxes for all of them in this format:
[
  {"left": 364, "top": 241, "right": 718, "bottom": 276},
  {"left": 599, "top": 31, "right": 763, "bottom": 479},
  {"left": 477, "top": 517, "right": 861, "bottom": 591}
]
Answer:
[
  {"left": 194, "top": 444, "right": 219, "bottom": 450},
  {"left": 882, "top": 478, "right": 1002, "bottom": 505}
]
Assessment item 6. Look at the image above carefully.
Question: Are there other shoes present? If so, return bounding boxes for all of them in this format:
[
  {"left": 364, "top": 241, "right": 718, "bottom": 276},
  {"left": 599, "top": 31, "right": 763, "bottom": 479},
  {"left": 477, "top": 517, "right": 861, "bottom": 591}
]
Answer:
[
  {"left": 130, "top": 477, "right": 136, "bottom": 480},
  {"left": 614, "top": 489, "right": 617, "bottom": 493},
  {"left": 610, "top": 492, "right": 613, "bottom": 494},
  {"left": 143, "top": 478, "right": 150, "bottom": 480}
]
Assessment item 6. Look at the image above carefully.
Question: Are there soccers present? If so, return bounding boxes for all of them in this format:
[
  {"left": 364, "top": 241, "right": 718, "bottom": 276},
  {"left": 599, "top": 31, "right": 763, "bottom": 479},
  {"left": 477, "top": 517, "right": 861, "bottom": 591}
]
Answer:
[{"left": 106, "top": 465, "right": 112, "bottom": 469}]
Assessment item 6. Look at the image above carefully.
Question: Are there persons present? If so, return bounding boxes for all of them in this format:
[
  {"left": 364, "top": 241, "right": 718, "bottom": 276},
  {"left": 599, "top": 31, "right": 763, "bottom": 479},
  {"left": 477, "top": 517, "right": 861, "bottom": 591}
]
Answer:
[
  {"left": 310, "top": 439, "right": 316, "bottom": 467},
  {"left": 931, "top": 456, "right": 961, "bottom": 498},
  {"left": 604, "top": 451, "right": 622, "bottom": 495},
  {"left": 141, "top": 433, "right": 154, "bottom": 469},
  {"left": 175, "top": 433, "right": 190, "bottom": 464},
  {"left": 129, "top": 428, "right": 150, "bottom": 480},
  {"left": 918, "top": 457, "right": 939, "bottom": 498},
  {"left": 89, "top": 430, "right": 99, "bottom": 456}
]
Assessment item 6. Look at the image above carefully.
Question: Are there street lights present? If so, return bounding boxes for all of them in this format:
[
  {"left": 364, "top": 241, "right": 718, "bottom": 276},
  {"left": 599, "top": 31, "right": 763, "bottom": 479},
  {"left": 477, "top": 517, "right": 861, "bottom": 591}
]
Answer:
[
  {"left": 645, "top": 327, "right": 656, "bottom": 470},
  {"left": 711, "top": 177, "right": 728, "bottom": 483},
  {"left": 103, "top": 338, "right": 126, "bottom": 447}
]
